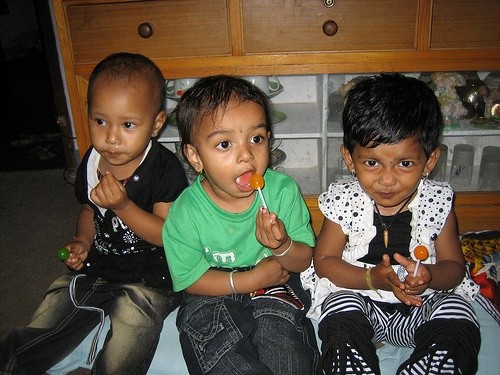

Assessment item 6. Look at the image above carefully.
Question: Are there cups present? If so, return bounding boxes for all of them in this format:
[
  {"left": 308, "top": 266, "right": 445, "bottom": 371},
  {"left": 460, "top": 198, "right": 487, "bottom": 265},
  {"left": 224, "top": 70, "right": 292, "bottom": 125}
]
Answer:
[
  {"left": 479, "top": 156, "right": 500, "bottom": 191},
  {"left": 266, "top": 138, "right": 354, "bottom": 180},
  {"left": 478, "top": 146, "right": 500, "bottom": 188},
  {"left": 448, "top": 144, "right": 475, "bottom": 189},
  {"left": 427, "top": 144, "right": 448, "bottom": 187},
  {"left": 174, "top": 141, "right": 199, "bottom": 186},
  {"left": 164, "top": 78, "right": 199, "bottom": 97},
  {"left": 239, "top": 75, "right": 281, "bottom": 95}
]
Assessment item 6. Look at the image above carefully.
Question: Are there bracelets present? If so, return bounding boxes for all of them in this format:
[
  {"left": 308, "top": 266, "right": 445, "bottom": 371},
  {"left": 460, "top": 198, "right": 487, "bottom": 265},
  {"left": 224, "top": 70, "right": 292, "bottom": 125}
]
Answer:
[
  {"left": 272, "top": 239, "right": 292, "bottom": 256},
  {"left": 366, "top": 268, "right": 378, "bottom": 291},
  {"left": 230, "top": 270, "right": 237, "bottom": 295}
]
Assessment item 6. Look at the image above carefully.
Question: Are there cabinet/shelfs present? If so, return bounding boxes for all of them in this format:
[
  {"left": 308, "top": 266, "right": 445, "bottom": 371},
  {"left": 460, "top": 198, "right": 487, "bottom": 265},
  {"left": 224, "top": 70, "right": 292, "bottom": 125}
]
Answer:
[{"left": 48, "top": 1, "right": 500, "bottom": 238}]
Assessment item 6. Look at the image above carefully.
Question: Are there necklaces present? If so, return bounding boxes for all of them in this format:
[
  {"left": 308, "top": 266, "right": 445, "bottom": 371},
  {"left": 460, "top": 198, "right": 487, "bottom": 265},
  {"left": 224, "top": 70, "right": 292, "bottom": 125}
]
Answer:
[{"left": 372, "top": 198, "right": 411, "bottom": 248}]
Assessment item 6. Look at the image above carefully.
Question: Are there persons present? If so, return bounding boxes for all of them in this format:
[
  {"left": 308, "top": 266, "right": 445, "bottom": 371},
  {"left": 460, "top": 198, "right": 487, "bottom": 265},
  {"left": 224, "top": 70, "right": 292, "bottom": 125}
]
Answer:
[
  {"left": 161, "top": 75, "right": 321, "bottom": 375},
  {"left": 0, "top": 52, "right": 190, "bottom": 375},
  {"left": 300, "top": 74, "right": 480, "bottom": 375}
]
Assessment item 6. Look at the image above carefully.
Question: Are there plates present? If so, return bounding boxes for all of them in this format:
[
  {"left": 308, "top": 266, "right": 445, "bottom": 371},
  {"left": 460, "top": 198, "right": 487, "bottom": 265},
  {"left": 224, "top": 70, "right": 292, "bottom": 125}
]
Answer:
[
  {"left": 268, "top": 147, "right": 286, "bottom": 169},
  {"left": 271, "top": 111, "right": 287, "bottom": 128},
  {"left": 417, "top": 72, "right": 500, "bottom": 118},
  {"left": 266, "top": 82, "right": 284, "bottom": 99},
  {"left": 164, "top": 86, "right": 182, "bottom": 101}
]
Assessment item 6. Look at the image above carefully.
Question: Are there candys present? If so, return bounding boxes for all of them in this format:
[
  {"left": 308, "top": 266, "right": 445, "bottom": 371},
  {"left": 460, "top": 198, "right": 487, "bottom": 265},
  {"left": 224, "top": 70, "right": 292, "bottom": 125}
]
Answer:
[
  {"left": 56, "top": 247, "right": 70, "bottom": 260},
  {"left": 249, "top": 173, "right": 267, "bottom": 208},
  {"left": 413, "top": 245, "right": 428, "bottom": 276}
]
